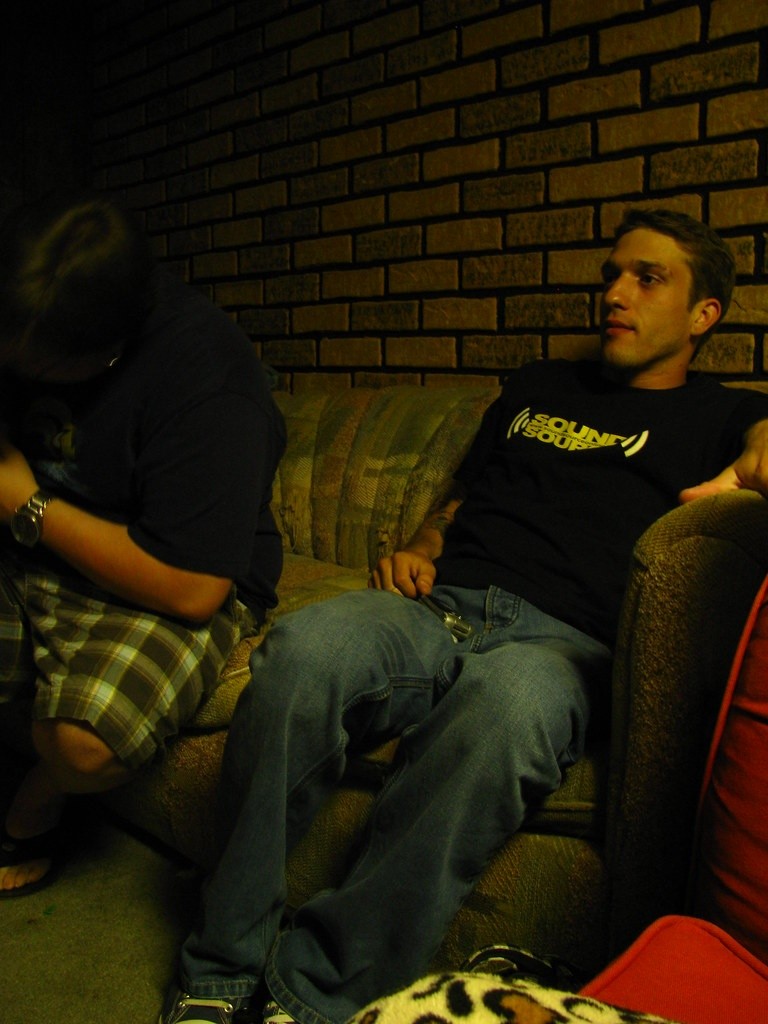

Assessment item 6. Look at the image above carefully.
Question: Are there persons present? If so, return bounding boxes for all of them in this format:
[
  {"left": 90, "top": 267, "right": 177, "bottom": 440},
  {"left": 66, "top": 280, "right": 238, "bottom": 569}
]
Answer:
[
  {"left": 0, "top": 198, "right": 283, "bottom": 899},
  {"left": 160, "top": 208, "right": 768, "bottom": 1024}
]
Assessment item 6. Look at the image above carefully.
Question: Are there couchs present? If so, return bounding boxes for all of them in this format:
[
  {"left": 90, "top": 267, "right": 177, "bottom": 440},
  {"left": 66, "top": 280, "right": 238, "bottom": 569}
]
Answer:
[{"left": 36, "top": 383, "right": 768, "bottom": 988}]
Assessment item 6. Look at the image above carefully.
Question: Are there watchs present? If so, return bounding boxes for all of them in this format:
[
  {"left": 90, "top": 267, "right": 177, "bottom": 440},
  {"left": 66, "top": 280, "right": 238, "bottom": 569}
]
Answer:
[{"left": 10, "top": 489, "right": 57, "bottom": 548}]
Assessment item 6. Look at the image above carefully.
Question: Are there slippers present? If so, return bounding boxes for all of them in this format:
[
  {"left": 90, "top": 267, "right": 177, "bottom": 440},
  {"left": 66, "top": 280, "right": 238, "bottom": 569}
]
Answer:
[{"left": 0, "top": 829, "right": 94, "bottom": 901}]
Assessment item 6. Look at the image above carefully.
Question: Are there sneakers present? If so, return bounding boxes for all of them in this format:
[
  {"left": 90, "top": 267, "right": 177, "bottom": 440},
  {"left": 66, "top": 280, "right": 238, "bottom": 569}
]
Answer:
[
  {"left": 159, "top": 967, "right": 243, "bottom": 1024},
  {"left": 264, "top": 999, "right": 294, "bottom": 1023}
]
